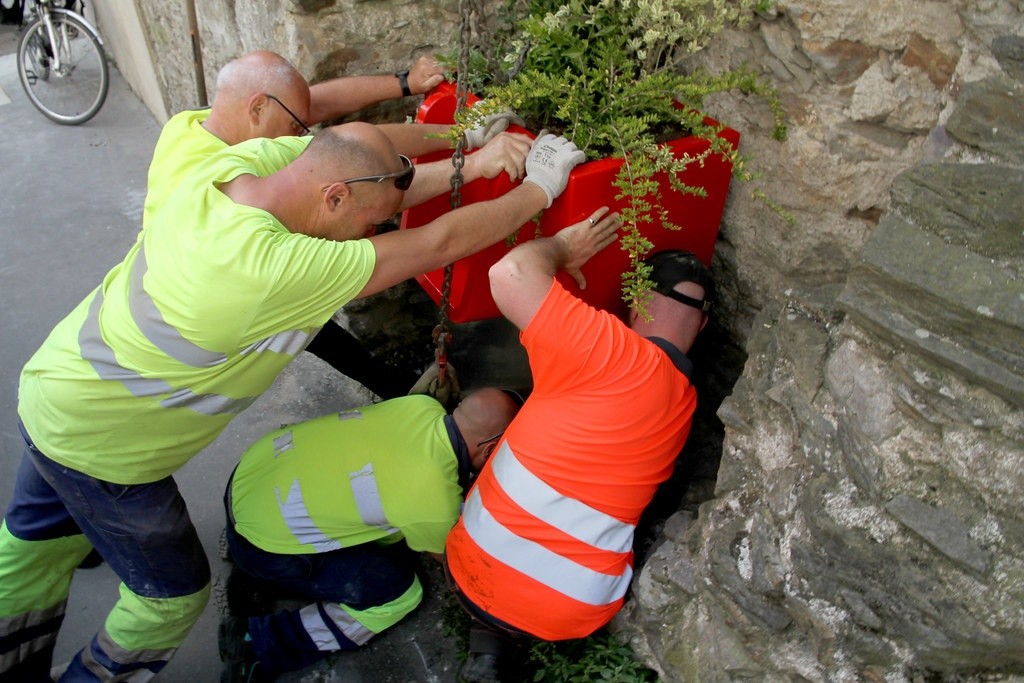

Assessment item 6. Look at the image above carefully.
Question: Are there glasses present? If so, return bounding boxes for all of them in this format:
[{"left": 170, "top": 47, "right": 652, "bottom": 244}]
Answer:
[
  {"left": 322, "top": 153, "right": 417, "bottom": 193},
  {"left": 266, "top": 95, "right": 311, "bottom": 137}
]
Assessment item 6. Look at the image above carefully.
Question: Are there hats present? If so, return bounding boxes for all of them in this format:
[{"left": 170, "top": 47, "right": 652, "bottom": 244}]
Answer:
[{"left": 643, "top": 248, "right": 714, "bottom": 311}]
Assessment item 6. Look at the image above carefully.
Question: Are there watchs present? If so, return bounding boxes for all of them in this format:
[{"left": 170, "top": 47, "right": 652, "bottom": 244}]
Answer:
[{"left": 395, "top": 70, "right": 413, "bottom": 96}]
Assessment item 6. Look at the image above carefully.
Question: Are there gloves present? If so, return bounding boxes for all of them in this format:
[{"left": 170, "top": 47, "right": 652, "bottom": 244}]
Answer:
[
  {"left": 461, "top": 100, "right": 530, "bottom": 152},
  {"left": 523, "top": 129, "right": 588, "bottom": 209}
]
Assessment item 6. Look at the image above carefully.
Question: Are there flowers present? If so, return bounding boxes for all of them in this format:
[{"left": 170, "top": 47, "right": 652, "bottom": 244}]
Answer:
[{"left": 417, "top": 1, "right": 798, "bottom": 326}]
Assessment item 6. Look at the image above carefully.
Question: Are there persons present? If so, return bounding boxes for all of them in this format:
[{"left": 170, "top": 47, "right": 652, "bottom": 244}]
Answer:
[
  {"left": 443, "top": 205, "right": 712, "bottom": 641},
  {"left": 0, "top": 97, "right": 585, "bottom": 683},
  {"left": 219, "top": 386, "right": 521, "bottom": 682},
  {"left": 74, "top": 50, "right": 534, "bottom": 571}
]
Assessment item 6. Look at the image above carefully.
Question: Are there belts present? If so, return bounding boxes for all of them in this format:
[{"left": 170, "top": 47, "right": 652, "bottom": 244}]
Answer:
[{"left": 442, "top": 548, "right": 528, "bottom": 660}]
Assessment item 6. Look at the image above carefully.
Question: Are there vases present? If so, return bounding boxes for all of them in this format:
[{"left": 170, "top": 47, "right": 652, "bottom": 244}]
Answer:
[{"left": 398, "top": 83, "right": 742, "bottom": 327}]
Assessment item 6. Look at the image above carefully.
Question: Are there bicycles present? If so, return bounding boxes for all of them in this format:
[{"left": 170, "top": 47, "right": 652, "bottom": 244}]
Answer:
[{"left": 15, "top": 0, "right": 109, "bottom": 127}]
